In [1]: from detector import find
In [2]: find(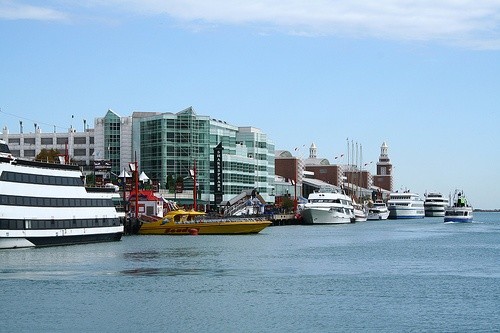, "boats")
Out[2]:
[444,189,474,223]
[387,192,426,219]
[425,194,449,217]
[367,202,390,219]
[138,210,273,234]
[352,204,367,222]
[302,192,356,225]
[0,140,127,249]
